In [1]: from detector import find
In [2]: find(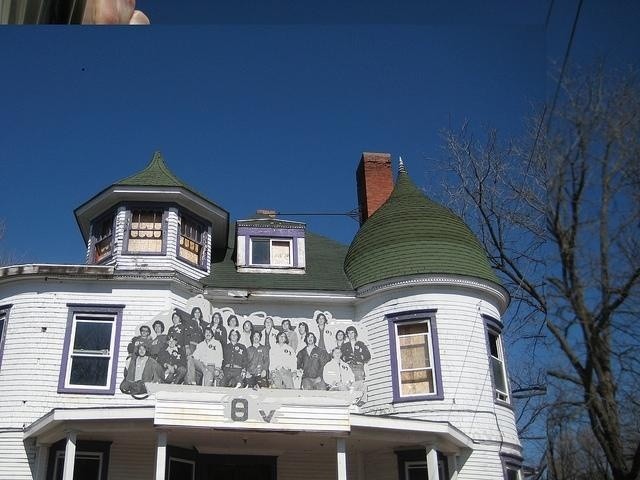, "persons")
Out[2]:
[114,308,371,390]
